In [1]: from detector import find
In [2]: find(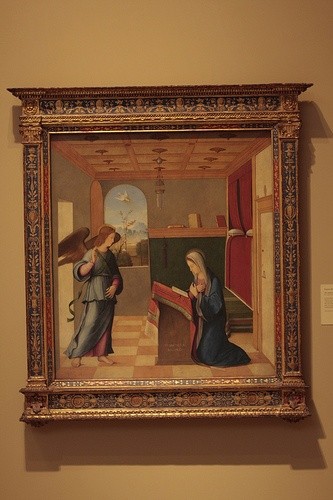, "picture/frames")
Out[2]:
[6,83,312,427]
